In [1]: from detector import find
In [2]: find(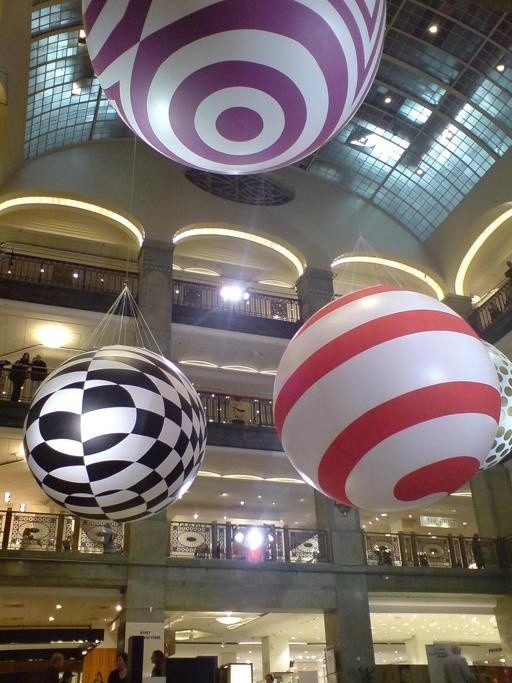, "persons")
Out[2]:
[44,652,66,683]
[443,643,478,683]
[27,354,50,395]
[150,649,166,677]
[195,541,210,558]
[100,522,115,554]
[61,670,77,683]
[470,533,487,570]
[8,352,31,400]
[107,652,130,683]
[265,674,273,683]
